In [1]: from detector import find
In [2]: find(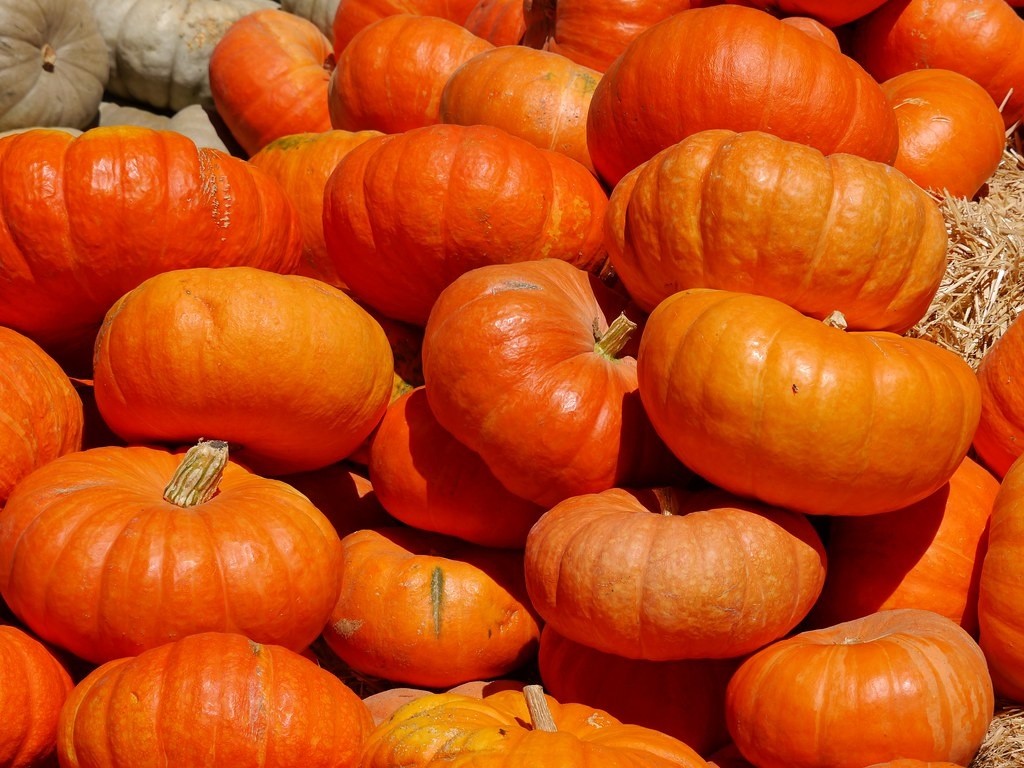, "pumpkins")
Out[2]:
[0,0,1024,768]
